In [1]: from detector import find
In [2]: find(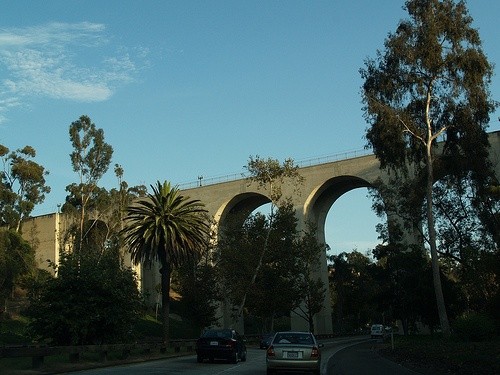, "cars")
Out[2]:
[371,324,386,338]
[260,333,275,349]
[264,331,324,375]
[194,328,248,363]
